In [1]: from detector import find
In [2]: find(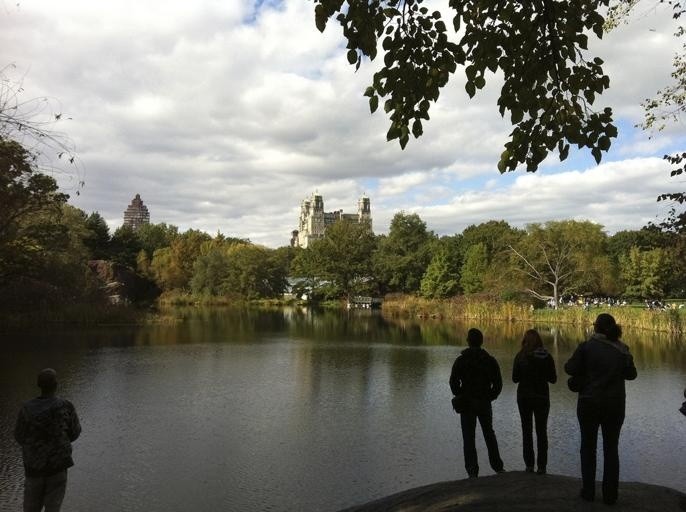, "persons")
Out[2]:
[511,328,557,475]
[544,293,666,311]
[566,312,638,505]
[449,328,507,480]
[14,365,82,512]
